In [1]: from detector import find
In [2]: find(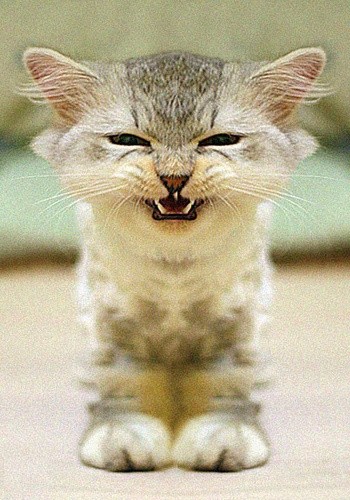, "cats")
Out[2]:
[12,44,336,471]
